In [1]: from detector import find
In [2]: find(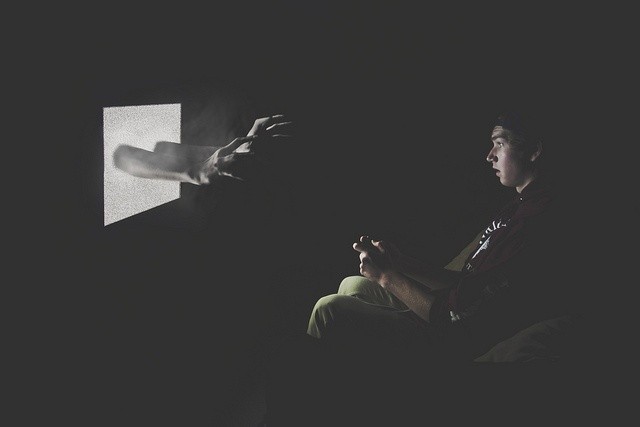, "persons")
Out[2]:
[306,114,576,345]
[113,113,293,187]
